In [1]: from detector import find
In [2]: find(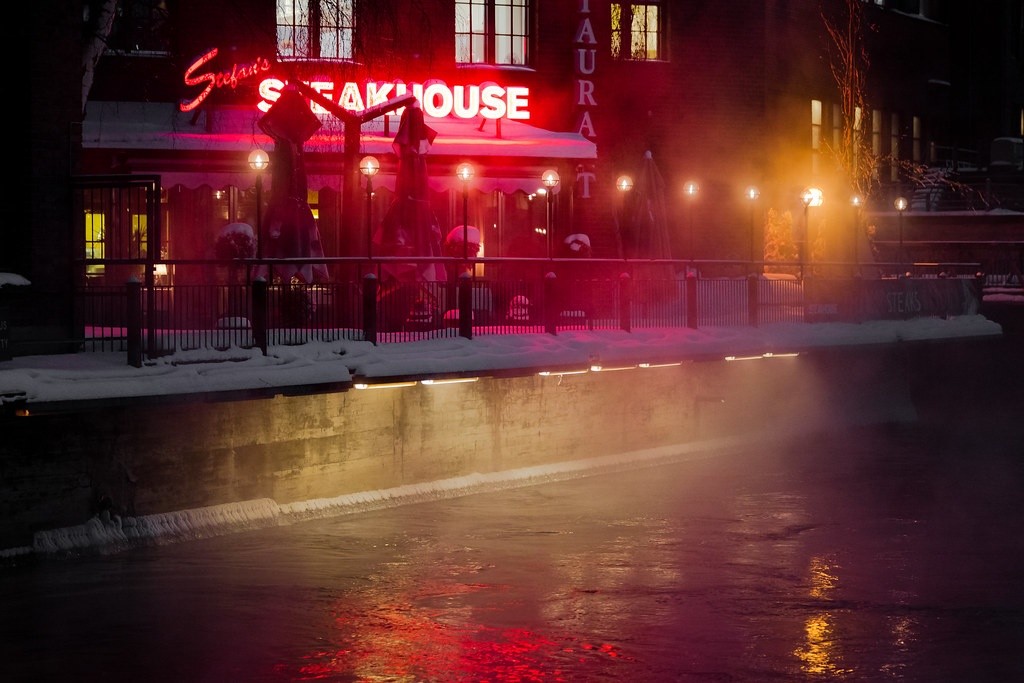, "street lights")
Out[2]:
[456,162,474,257]
[895,197,908,263]
[249,149,270,276]
[684,181,698,261]
[616,175,634,258]
[543,170,560,257]
[746,186,761,259]
[850,194,863,262]
[359,155,380,272]
